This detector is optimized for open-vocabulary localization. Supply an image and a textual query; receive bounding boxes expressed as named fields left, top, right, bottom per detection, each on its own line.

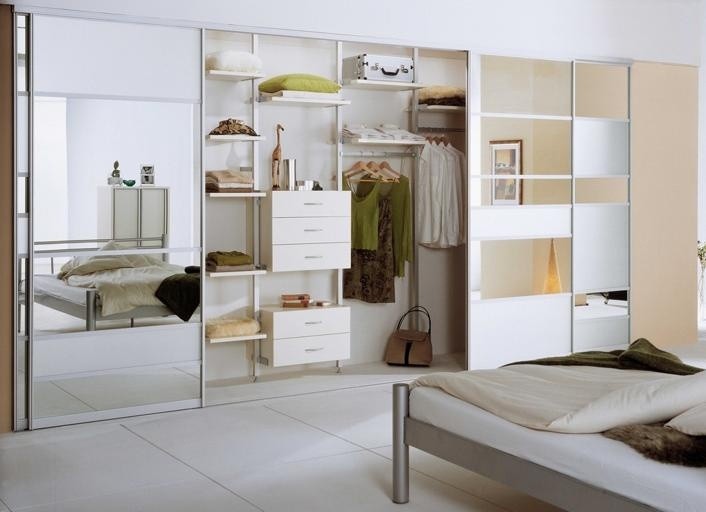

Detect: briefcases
left=342, top=54, right=414, bottom=82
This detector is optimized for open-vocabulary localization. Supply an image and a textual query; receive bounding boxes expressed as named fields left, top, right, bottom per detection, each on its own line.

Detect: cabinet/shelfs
left=97, top=185, right=170, bottom=267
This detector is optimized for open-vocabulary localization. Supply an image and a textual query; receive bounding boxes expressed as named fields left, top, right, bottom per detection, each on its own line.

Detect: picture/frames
left=490, top=140, right=524, bottom=208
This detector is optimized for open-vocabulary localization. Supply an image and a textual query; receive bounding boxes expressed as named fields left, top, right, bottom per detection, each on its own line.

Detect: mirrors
left=30, top=96, right=202, bottom=418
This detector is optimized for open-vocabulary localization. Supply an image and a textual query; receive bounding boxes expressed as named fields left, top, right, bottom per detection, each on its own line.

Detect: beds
left=29, top=238, right=199, bottom=330
left=392, top=339, right=705, bottom=512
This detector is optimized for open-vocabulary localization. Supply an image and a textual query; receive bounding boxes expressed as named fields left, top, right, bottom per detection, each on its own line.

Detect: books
left=282, top=297, right=313, bottom=304
left=310, top=299, right=332, bottom=306
left=282, top=303, right=314, bottom=308
left=280, top=294, right=310, bottom=300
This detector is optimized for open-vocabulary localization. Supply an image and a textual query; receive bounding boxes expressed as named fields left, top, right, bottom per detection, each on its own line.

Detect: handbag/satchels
left=385, top=307, right=432, bottom=367
